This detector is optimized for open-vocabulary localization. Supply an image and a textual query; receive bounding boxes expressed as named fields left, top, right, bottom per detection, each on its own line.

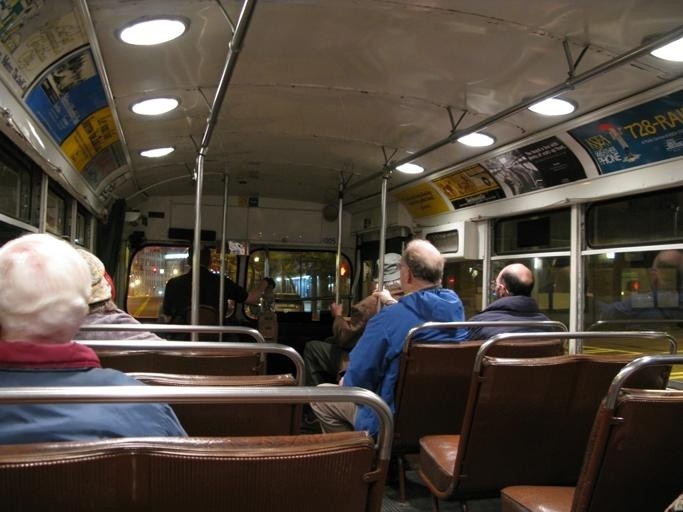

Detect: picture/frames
left=24, top=45, right=128, bottom=191
left=394, top=182, right=450, bottom=220
left=431, top=164, right=508, bottom=211
left=482, top=136, right=588, bottom=197
left=566, top=90, right=683, bottom=176
left=0, top=1, right=90, bottom=98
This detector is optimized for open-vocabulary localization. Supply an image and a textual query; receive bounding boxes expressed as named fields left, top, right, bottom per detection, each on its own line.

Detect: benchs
left=76, top=337, right=305, bottom=437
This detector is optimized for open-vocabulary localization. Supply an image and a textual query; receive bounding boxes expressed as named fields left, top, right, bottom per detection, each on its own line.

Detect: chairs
left=499, top=358, right=682, bottom=512
left=418, top=330, right=676, bottom=512
left=80, top=318, right=271, bottom=374
left=0, top=383, right=396, bottom=509
left=182, top=304, right=219, bottom=343
left=393, top=321, right=577, bottom=504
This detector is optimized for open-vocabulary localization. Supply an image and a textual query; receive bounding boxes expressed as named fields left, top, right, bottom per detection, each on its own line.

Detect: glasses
left=490, top=280, right=509, bottom=297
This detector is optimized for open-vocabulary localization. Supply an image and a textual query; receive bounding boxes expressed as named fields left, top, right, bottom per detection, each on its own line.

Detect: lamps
left=394, top=162, right=423, bottom=177
left=129, top=93, right=181, bottom=115
left=139, top=144, right=176, bottom=158
left=455, top=129, right=495, bottom=149
left=114, top=15, right=190, bottom=46
left=524, top=94, right=578, bottom=117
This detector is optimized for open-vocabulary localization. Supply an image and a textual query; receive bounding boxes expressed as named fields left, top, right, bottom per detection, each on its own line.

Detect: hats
left=75, top=248, right=113, bottom=305
left=373, top=253, right=402, bottom=283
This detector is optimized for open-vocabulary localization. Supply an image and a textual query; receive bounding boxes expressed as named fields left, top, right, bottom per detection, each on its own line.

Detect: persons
left=302, top=253, right=404, bottom=387
left=162, top=243, right=262, bottom=341
left=0, top=233, right=190, bottom=445
left=593, top=249, right=683, bottom=333
left=75, top=247, right=161, bottom=341
left=467, top=263, right=556, bottom=340
left=307, top=239, right=467, bottom=447
